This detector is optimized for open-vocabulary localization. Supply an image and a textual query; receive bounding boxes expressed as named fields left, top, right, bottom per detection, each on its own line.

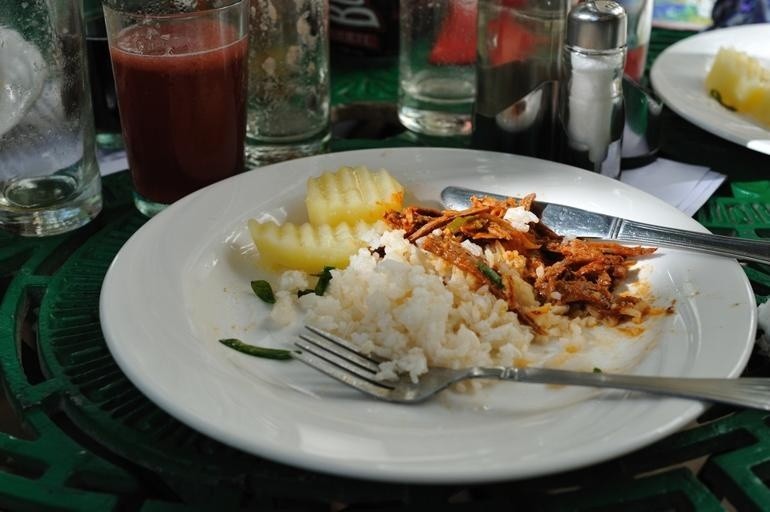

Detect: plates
left=99, top=145, right=757, bottom=484
left=649, top=22, right=770, bottom=156
left=0, top=29, right=47, bottom=137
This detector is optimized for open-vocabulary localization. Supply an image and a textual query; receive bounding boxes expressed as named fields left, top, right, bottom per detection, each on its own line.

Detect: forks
left=291, top=325, right=770, bottom=411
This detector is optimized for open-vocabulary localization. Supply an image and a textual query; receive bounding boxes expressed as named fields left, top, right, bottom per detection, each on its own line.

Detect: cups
left=102, top=0, right=250, bottom=219
left=397, top=0, right=569, bottom=160
left=0, top=0, right=103, bottom=237
left=244, top=0, right=333, bottom=170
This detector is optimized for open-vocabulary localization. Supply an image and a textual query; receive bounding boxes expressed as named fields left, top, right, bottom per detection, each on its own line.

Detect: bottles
left=567, top=0, right=653, bottom=181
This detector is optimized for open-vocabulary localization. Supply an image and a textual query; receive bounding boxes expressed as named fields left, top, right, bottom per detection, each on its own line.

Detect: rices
left=271, top=207, right=598, bottom=395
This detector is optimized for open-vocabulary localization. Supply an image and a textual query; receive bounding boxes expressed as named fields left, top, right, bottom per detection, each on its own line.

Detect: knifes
left=439, top=186, right=770, bottom=265
